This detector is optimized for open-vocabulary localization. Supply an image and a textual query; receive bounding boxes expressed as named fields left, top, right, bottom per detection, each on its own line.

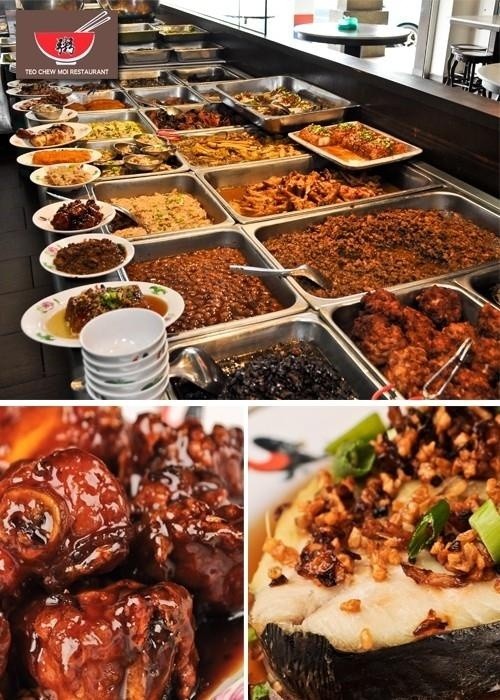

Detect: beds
left=293, top=23, right=411, bottom=58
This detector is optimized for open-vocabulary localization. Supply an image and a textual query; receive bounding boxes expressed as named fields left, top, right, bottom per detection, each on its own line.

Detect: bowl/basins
left=92, top=131, right=177, bottom=171
left=81, top=307, right=170, bottom=400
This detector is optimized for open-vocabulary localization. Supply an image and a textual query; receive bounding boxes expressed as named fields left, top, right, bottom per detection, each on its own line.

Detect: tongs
left=422, top=337, right=477, bottom=399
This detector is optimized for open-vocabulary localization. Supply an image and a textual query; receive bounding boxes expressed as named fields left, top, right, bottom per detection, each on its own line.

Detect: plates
left=28, top=162, right=102, bottom=189
left=5, top=77, right=77, bottom=124
left=9, top=122, right=92, bottom=151
left=34, top=229, right=134, bottom=278
left=31, top=200, right=117, bottom=235
left=19, top=277, right=191, bottom=351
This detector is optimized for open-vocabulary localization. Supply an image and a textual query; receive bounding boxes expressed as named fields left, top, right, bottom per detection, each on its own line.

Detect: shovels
left=230, top=264, right=329, bottom=290
left=135, top=98, right=183, bottom=115
left=236, top=104, right=289, bottom=113
left=46, top=192, right=138, bottom=226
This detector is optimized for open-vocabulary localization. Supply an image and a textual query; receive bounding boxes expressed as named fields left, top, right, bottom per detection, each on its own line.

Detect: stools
left=477, top=63, right=500, bottom=101
left=444, top=44, right=478, bottom=85
left=455, top=51, right=493, bottom=92
left=451, top=46, right=486, bottom=87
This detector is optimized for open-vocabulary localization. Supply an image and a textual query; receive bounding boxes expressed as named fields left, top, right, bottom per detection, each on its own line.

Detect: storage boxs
left=329, top=10, right=388, bottom=57
left=337, top=0, right=383, bottom=10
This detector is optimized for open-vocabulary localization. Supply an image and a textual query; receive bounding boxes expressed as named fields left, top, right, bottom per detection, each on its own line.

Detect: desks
left=450, top=15, right=500, bottom=32
